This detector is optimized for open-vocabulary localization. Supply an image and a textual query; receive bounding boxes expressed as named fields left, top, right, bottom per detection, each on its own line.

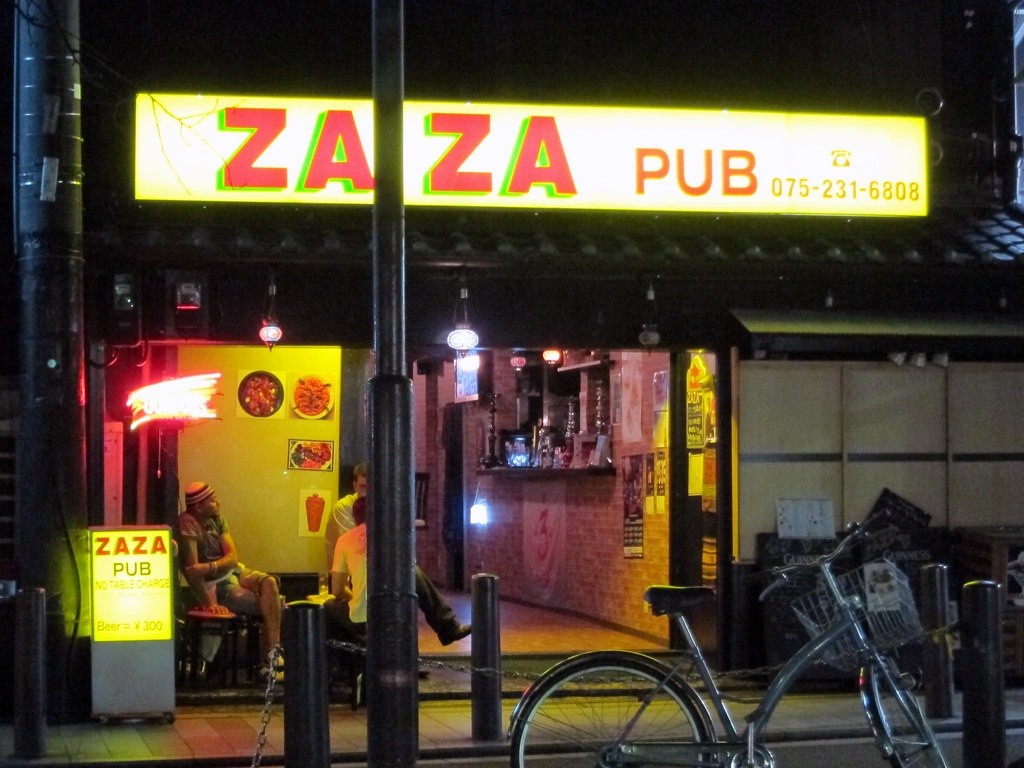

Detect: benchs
left=268, top=570, right=320, bottom=605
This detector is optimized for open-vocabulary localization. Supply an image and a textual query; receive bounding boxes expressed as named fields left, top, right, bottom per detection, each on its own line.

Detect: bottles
left=305, top=494, right=325, bottom=532
left=553, top=447, right=562, bottom=468
left=541, top=449, right=552, bottom=468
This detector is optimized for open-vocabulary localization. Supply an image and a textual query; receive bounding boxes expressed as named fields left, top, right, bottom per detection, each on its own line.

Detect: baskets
left=789, top=558, right=922, bottom=671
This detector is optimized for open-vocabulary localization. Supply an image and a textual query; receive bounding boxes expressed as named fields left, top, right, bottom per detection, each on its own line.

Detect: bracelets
left=208, top=561, right=218, bottom=579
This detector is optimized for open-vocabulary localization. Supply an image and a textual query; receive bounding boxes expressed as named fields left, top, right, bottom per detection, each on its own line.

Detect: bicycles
left=506, top=508, right=948, bottom=768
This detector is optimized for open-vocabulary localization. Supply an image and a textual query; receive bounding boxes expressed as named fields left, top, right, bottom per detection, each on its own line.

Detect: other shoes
left=441, top=624, right=472, bottom=646
left=418, top=669, right=429, bottom=677
left=266, top=649, right=284, bottom=668
left=259, top=665, right=284, bottom=681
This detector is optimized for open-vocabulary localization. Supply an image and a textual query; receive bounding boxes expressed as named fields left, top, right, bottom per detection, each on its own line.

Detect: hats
left=185, top=482, right=214, bottom=505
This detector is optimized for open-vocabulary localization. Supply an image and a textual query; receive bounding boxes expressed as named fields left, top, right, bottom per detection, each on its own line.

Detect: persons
left=322, top=495, right=366, bottom=671
left=177, top=481, right=286, bottom=682
left=324, top=462, right=472, bottom=646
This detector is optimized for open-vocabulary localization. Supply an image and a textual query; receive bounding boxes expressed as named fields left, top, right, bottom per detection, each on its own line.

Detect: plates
left=290, top=374, right=334, bottom=419
left=238, top=371, right=283, bottom=416
left=290, top=442, right=331, bottom=469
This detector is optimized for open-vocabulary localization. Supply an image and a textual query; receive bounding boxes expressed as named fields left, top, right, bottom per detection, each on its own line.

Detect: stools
left=186, top=609, right=240, bottom=688
left=346, top=628, right=368, bottom=711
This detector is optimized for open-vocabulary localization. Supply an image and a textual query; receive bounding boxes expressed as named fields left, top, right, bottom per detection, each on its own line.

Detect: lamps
left=640, top=288, right=661, bottom=355
left=257, top=283, right=283, bottom=347
left=446, top=264, right=480, bottom=355
left=175, top=264, right=206, bottom=310
